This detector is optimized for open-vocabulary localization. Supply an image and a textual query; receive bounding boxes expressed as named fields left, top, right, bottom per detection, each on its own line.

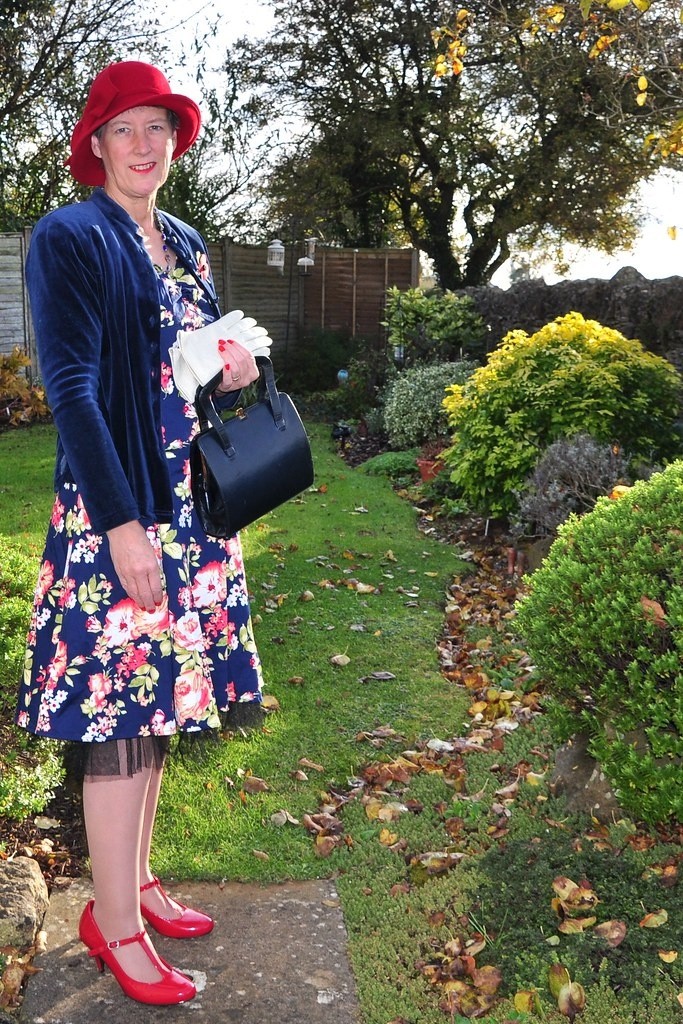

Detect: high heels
left=78, top=899, right=197, bottom=1007
left=139, top=875, right=215, bottom=939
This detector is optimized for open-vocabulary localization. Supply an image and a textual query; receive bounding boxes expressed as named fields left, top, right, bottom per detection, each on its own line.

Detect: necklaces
left=153, top=215, right=171, bottom=273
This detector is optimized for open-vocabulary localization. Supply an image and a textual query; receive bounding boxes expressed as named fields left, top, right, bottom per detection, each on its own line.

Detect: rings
left=233, top=375, right=240, bottom=381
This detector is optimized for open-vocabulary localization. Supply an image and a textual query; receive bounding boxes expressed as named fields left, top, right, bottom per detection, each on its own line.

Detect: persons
left=14, top=62, right=267, bottom=1005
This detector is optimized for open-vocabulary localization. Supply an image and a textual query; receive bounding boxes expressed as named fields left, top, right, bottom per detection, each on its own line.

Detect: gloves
left=176, top=309, right=273, bottom=388
left=167, top=346, right=201, bottom=405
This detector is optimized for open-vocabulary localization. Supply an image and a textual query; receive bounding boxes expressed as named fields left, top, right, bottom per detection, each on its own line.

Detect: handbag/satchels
left=189, top=355, right=315, bottom=541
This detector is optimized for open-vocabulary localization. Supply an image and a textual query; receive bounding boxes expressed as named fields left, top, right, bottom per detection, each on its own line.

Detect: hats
left=63, top=60, right=202, bottom=186
